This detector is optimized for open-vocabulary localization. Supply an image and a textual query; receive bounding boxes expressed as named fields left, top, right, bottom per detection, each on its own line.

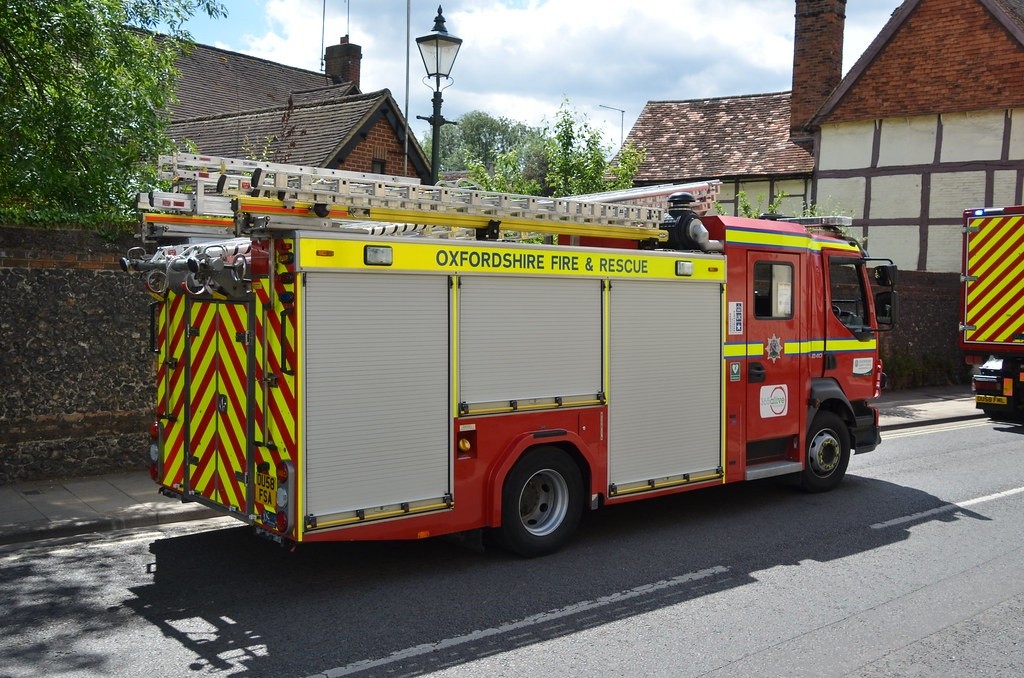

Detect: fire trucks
left=117, top=148, right=899, bottom=558
left=958, top=205, right=1024, bottom=420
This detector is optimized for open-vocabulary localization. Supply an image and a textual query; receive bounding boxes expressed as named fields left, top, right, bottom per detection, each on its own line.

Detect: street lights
left=415, top=4, right=464, bottom=188
